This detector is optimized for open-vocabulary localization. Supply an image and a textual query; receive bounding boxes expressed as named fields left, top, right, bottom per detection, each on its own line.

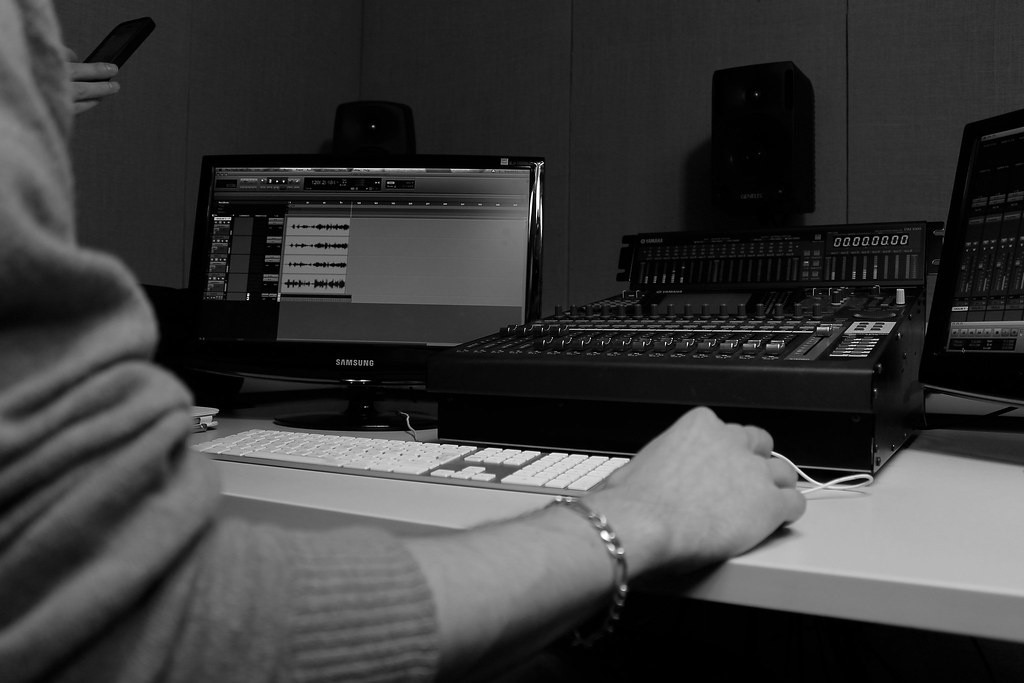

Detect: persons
left=0, top=0, right=807, bottom=683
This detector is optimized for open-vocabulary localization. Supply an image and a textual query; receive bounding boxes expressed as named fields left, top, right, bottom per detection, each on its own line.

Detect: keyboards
left=192, top=428, right=632, bottom=498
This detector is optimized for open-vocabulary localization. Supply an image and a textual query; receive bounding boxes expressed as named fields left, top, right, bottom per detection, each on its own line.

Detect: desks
left=190, top=379, right=1024, bottom=643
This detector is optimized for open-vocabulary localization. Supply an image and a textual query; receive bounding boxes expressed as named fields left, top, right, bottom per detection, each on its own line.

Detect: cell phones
left=81, top=17, right=157, bottom=82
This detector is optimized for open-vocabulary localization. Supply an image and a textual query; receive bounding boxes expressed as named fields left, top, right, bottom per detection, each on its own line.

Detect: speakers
left=713, top=60, right=818, bottom=214
left=336, top=100, right=418, bottom=158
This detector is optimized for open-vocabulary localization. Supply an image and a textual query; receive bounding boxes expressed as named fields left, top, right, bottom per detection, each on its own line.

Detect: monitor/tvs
left=919, top=110, right=1024, bottom=407
left=184, top=156, right=547, bottom=431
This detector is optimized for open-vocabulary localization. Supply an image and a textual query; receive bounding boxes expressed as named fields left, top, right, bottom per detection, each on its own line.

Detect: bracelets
left=544, top=497, right=629, bottom=647
